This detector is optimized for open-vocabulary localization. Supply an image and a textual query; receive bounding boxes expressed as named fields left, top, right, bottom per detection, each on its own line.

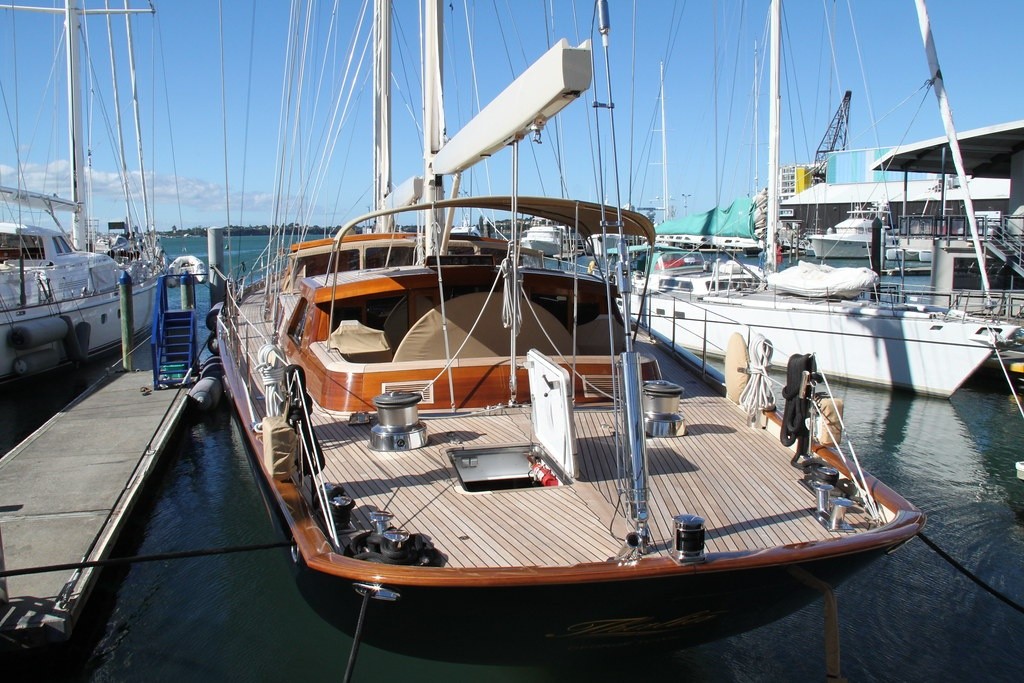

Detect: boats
left=806, top=205, right=883, bottom=260
left=513, top=213, right=578, bottom=261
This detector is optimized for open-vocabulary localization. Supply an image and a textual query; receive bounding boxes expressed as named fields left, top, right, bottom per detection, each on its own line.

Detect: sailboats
left=198, top=0, right=923, bottom=604
left=617, top=0, right=1017, bottom=401
left=0, top=0, right=173, bottom=392
